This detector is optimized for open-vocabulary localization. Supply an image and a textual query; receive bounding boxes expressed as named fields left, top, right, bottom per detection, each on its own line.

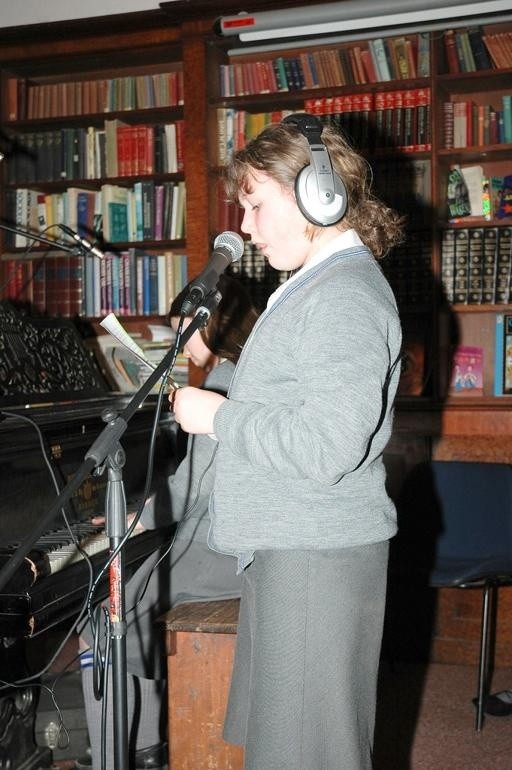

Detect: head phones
left=281, top=113, right=347, bottom=227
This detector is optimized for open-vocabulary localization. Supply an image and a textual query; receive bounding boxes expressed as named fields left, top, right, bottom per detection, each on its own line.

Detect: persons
left=164, top=108, right=401, bottom=770
left=68, top=267, right=267, bottom=770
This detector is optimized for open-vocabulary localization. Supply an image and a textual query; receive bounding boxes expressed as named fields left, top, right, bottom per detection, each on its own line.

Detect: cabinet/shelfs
left=2, top=20, right=207, bottom=389
left=209, top=32, right=511, bottom=463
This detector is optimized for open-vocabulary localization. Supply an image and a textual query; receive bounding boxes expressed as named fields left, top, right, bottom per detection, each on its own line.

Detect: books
left=1, top=71, right=189, bottom=182
left=397, top=313, right=512, bottom=398
left=217, top=174, right=298, bottom=287
left=440, top=28, right=509, bottom=306
left=98, top=326, right=189, bottom=392
left=375, top=157, right=434, bottom=305
left=218, top=34, right=431, bottom=164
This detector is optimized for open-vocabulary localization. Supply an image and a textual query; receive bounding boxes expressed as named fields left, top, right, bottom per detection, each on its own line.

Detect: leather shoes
left=74, top=743, right=164, bottom=770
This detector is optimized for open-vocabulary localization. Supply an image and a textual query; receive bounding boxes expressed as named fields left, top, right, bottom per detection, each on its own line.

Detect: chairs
left=405, top=464, right=510, bottom=732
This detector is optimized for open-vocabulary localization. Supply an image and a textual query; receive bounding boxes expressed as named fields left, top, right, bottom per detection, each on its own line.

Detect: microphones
left=59, top=223, right=105, bottom=259
left=181, top=230, right=244, bottom=315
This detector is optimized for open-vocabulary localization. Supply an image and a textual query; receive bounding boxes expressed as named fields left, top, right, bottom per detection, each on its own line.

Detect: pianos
left=1, top=298, right=189, bottom=770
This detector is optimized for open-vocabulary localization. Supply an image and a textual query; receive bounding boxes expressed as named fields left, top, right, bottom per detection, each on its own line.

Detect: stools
left=152, top=597, right=245, bottom=770
